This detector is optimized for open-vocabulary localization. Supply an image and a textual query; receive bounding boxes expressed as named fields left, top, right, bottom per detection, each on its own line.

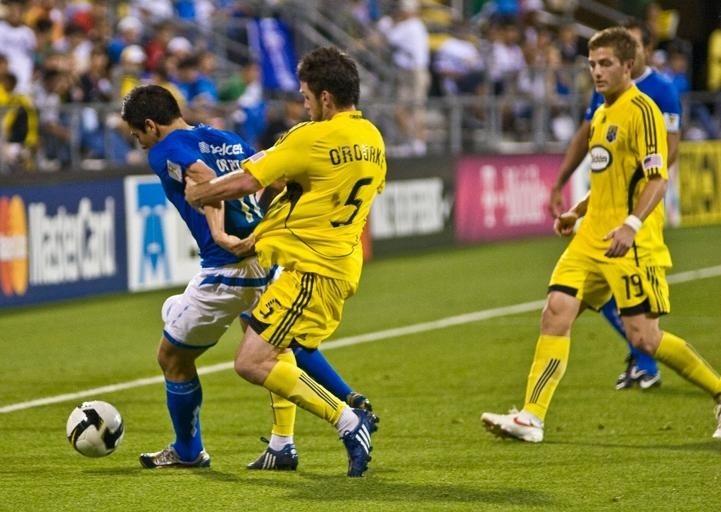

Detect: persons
left=479, top=22, right=721, bottom=446
left=547, top=17, right=685, bottom=394
left=181, top=44, right=389, bottom=479
left=0, top=1, right=721, bottom=178
left=121, top=82, right=376, bottom=470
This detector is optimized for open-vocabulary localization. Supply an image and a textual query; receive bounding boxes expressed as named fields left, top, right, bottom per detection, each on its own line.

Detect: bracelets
left=621, top=213, right=644, bottom=234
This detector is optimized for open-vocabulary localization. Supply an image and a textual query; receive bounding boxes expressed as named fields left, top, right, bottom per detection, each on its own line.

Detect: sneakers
left=340, top=407, right=380, bottom=477
left=481, top=408, right=544, bottom=443
left=139, top=443, right=211, bottom=468
left=712, top=411, right=721, bottom=439
left=246, top=444, right=298, bottom=471
left=349, top=393, right=372, bottom=413
left=615, top=366, right=662, bottom=392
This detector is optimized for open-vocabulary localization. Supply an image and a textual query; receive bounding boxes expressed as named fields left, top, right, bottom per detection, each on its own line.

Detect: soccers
left=66, top=401, right=124, bottom=457
